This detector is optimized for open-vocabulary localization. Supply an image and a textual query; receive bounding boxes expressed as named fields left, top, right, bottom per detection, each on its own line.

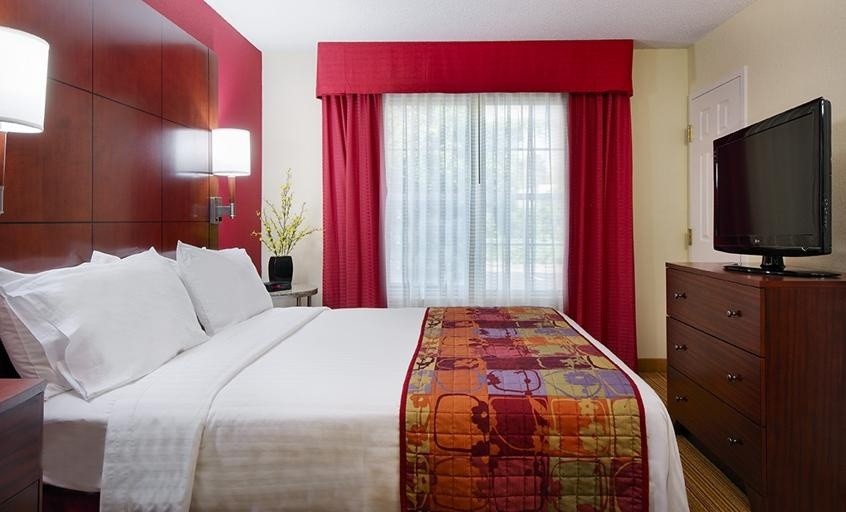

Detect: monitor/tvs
left=712, top=95, right=843, bottom=277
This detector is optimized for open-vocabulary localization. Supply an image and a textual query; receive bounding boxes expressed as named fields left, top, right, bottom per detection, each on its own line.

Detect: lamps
left=0, top=25, right=50, bottom=134
left=210, top=127, right=251, bottom=224
left=174, top=127, right=212, bottom=175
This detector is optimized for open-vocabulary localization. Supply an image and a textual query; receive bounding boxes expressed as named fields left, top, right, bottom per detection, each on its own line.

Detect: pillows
left=0, top=249, right=123, bottom=402
left=176, top=239, right=274, bottom=337
left=0, top=247, right=210, bottom=401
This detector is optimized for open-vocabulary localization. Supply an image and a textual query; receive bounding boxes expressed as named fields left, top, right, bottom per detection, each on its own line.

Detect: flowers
left=250, top=166, right=324, bottom=256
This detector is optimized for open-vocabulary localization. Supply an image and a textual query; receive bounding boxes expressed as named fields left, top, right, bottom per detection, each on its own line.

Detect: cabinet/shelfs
left=664, top=260, right=845, bottom=512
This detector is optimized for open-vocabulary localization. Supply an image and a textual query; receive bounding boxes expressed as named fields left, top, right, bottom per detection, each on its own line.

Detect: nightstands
left=1, top=377, right=48, bottom=512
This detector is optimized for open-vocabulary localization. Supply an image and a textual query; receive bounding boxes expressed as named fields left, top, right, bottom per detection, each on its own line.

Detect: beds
left=40, top=304, right=691, bottom=512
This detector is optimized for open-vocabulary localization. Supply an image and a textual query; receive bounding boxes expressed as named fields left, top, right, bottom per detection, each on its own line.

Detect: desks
left=268, top=283, right=318, bottom=307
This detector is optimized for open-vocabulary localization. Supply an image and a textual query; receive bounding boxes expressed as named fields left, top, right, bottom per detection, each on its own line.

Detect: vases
left=267, top=257, right=293, bottom=291
